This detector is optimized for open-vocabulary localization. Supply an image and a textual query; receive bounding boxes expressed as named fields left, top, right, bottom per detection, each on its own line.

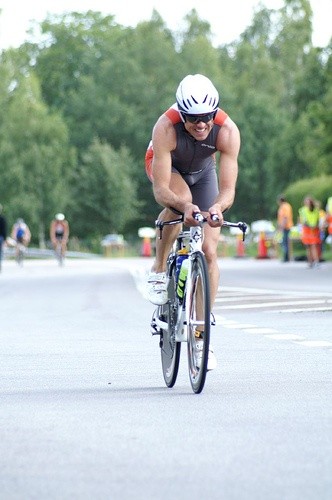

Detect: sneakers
left=147, top=268, right=168, bottom=305
left=195, top=339, right=218, bottom=370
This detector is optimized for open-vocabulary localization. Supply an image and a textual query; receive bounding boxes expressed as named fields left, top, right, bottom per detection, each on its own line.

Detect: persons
left=144, top=73, right=241, bottom=371
left=0, top=195, right=332, bottom=271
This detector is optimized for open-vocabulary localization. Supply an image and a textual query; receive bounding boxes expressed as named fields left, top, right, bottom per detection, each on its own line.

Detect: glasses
left=183, top=113, right=215, bottom=123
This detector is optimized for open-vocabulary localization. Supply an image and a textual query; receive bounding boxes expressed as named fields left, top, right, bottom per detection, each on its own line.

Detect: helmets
left=56, top=213, right=65, bottom=221
left=176, top=74, right=220, bottom=123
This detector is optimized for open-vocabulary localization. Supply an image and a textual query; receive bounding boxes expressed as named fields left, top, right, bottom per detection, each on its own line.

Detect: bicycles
left=13, top=239, right=28, bottom=265
left=150, top=212, right=248, bottom=394
left=51, top=238, right=70, bottom=274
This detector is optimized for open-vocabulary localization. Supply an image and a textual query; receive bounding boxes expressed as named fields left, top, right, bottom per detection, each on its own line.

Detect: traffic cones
left=101, top=245, right=113, bottom=262
left=140, top=235, right=156, bottom=259
left=229, top=228, right=247, bottom=261
left=255, top=231, right=270, bottom=262
left=113, top=241, right=126, bottom=259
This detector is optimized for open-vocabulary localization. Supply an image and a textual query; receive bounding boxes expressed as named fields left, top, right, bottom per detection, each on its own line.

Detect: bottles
left=176, top=249, right=189, bottom=283
left=177, top=255, right=192, bottom=297
